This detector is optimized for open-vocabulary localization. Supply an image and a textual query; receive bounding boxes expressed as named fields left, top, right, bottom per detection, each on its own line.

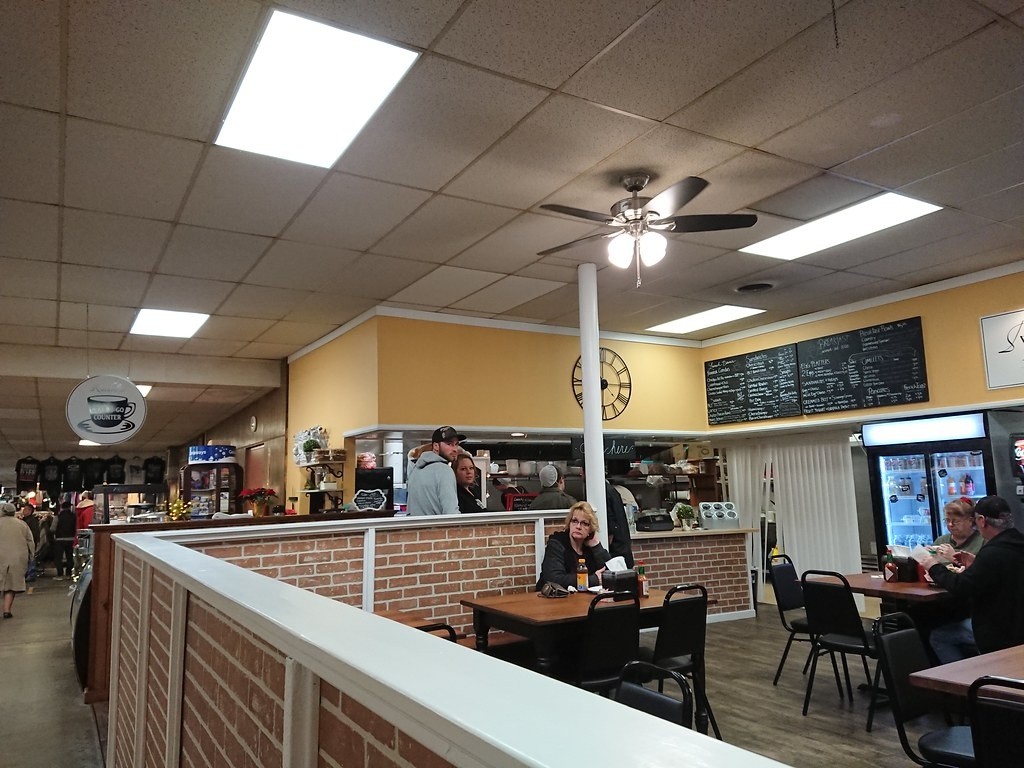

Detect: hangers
left=19, top=452, right=165, bottom=462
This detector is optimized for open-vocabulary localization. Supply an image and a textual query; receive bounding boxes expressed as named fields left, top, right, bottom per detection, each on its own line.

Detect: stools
left=394, top=615, right=466, bottom=640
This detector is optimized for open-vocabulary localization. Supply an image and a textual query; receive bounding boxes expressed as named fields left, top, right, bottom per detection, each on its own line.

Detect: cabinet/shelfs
left=421, top=437, right=717, bottom=518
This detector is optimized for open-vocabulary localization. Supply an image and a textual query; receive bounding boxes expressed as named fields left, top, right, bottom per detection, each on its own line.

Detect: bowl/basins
left=505, top=459, right=581, bottom=477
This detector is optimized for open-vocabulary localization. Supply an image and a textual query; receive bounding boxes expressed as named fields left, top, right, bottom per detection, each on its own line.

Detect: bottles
left=576, top=559, right=588, bottom=593
left=887, top=474, right=975, bottom=496
left=894, top=533, right=933, bottom=551
left=636, top=561, right=650, bottom=599
left=884, top=550, right=897, bottom=583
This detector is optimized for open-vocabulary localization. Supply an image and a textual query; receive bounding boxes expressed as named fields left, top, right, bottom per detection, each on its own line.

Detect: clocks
left=571, top=347, right=632, bottom=421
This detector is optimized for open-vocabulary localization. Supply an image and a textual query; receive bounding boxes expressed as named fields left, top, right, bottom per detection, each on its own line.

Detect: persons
left=50, top=502, right=77, bottom=580
left=451, top=454, right=487, bottom=514
left=72, top=491, right=94, bottom=546
left=528, top=464, right=577, bottom=510
left=919, top=495, right=1024, bottom=665
left=492, top=478, right=528, bottom=509
left=536, top=501, right=612, bottom=591
left=407, top=426, right=467, bottom=516
left=0, top=503, right=35, bottom=618
left=579, top=461, right=634, bottom=569
left=21, top=503, right=41, bottom=594
left=924, top=497, right=990, bottom=568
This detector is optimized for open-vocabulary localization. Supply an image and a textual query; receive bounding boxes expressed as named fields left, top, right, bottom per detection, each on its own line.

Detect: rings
left=941, top=550, right=943, bottom=554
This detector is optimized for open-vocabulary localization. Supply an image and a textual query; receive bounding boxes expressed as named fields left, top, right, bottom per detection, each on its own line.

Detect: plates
left=588, top=585, right=604, bottom=593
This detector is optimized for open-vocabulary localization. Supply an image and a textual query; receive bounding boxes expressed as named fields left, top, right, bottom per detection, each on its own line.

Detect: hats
left=966, top=495, right=1011, bottom=521
left=4, top=503, right=16, bottom=512
left=538, top=465, right=558, bottom=487
left=62, top=502, right=73, bottom=508
left=432, top=426, right=466, bottom=443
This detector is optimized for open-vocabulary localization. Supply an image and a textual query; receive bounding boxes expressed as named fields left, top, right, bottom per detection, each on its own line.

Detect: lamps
left=609, top=196, right=667, bottom=270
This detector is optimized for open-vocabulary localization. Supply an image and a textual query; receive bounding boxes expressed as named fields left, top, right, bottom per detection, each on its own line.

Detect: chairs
left=769, top=553, right=1024, bottom=768
left=548, top=584, right=724, bottom=741
left=414, top=623, right=456, bottom=642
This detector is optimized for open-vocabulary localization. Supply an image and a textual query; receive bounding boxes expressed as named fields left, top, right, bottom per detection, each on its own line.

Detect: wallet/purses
left=537, top=581, right=569, bottom=597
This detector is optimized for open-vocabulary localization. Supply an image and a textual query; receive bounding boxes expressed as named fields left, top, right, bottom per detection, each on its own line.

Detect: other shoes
left=67, top=575, right=71, bottom=579
left=53, top=575, right=64, bottom=580
left=3, top=611, right=13, bottom=618
left=25, top=575, right=36, bottom=582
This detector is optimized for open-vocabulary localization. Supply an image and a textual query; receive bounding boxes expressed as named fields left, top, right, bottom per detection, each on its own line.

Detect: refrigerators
left=861, top=409, right=1024, bottom=572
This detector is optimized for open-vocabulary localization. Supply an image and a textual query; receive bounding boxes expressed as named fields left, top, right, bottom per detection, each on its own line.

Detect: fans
left=536, top=172, right=759, bottom=288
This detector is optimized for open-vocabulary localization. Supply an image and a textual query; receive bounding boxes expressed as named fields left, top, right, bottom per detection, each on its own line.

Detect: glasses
left=570, top=517, right=591, bottom=529
left=943, top=517, right=970, bottom=525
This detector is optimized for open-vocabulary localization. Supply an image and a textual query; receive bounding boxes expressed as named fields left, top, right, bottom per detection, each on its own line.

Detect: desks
left=794, top=572, right=958, bottom=695
left=909, top=644, right=1024, bottom=703
left=460, top=588, right=719, bottom=736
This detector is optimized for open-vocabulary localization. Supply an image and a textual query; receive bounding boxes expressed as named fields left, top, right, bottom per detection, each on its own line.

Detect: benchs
left=456, top=629, right=530, bottom=658
